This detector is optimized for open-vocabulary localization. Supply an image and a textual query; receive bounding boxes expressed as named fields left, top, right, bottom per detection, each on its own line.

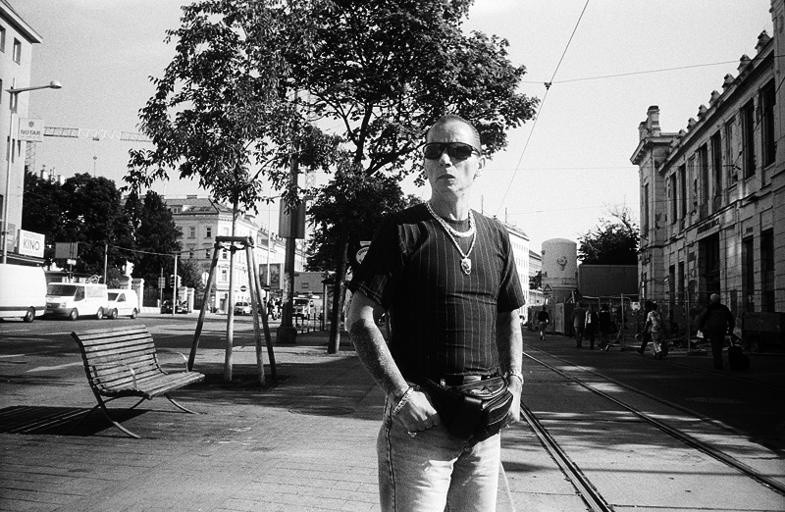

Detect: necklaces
left=425, top=200, right=477, bottom=276
left=427, top=201, right=475, bottom=237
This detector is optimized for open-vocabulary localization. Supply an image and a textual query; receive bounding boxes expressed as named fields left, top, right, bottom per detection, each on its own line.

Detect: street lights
left=1, top=80, right=62, bottom=265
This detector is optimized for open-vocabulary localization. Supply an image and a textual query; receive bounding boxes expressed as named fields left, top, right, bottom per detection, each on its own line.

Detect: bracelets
left=504, top=370, right=524, bottom=385
left=392, top=387, right=414, bottom=417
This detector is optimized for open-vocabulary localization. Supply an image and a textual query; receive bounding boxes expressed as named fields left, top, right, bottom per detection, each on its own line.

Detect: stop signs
left=240, top=286, right=246, bottom=291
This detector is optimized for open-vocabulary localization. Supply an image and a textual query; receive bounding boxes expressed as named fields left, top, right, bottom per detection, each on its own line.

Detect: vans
left=0, top=263, right=49, bottom=322
left=46, top=279, right=109, bottom=320
left=291, top=297, right=315, bottom=315
left=109, top=288, right=140, bottom=319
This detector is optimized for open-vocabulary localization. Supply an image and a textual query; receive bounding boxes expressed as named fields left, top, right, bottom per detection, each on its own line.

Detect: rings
left=408, top=432, right=418, bottom=438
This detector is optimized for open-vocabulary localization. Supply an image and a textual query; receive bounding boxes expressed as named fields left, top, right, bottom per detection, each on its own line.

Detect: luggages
left=729, top=347, right=749, bottom=370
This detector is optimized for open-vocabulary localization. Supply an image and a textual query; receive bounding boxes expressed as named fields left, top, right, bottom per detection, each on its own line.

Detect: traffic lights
left=170, top=274, right=181, bottom=288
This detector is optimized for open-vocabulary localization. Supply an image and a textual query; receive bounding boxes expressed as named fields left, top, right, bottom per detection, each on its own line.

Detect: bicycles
left=539, top=324, right=545, bottom=340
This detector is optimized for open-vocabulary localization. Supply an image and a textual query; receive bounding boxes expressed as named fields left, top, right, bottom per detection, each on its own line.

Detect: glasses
left=423, top=142, right=480, bottom=160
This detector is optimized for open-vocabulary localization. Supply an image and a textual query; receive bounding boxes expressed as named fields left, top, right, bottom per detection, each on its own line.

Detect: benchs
left=70, top=324, right=206, bottom=440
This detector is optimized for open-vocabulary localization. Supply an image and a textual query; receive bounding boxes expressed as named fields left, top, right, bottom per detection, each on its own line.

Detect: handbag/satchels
left=425, top=375, right=513, bottom=440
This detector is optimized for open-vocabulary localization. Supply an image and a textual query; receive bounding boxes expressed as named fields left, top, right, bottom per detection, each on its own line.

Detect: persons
left=570, top=300, right=668, bottom=359
left=696, top=293, right=735, bottom=370
left=266, top=297, right=283, bottom=320
left=346, top=115, right=527, bottom=512
left=537, top=307, right=552, bottom=341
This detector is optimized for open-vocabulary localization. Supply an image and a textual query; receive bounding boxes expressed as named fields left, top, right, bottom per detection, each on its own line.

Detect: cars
left=235, top=301, right=253, bottom=315
left=161, top=298, right=189, bottom=313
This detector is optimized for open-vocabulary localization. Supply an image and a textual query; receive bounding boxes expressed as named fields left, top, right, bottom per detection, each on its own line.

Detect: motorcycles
left=648, top=333, right=668, bottom=359
